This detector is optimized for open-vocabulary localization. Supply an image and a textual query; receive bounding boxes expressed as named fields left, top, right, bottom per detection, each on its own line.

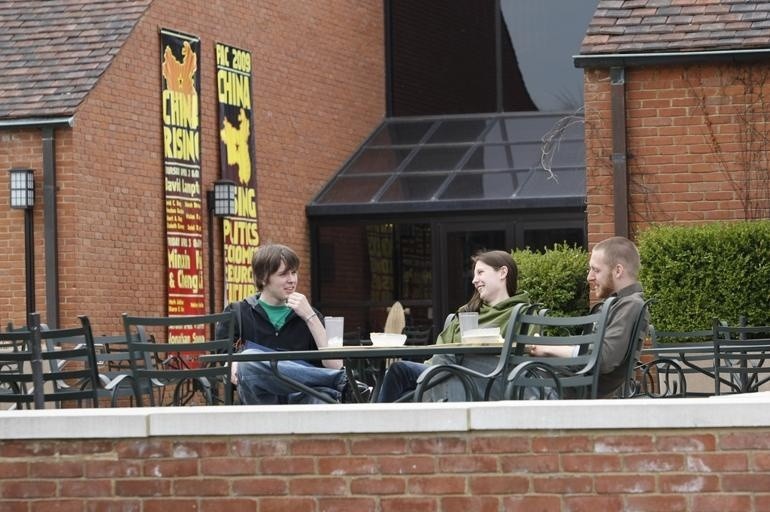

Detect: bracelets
left=304, top=312, right=318, bottom=323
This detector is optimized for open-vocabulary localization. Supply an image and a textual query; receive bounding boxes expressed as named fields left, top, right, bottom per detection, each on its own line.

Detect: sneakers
left=343, top=380, right=373, bottom=401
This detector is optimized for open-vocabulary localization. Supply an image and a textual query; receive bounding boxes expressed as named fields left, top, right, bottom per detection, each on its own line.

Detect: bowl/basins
left=370, top=332, right=407, bottom=347
left=461, top=328, right=501, bottom=344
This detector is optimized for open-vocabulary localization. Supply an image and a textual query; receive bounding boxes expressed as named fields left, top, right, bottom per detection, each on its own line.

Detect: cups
left=323, top=316, right=344, bottom=348
left=457, top=313, right=479, bottom=335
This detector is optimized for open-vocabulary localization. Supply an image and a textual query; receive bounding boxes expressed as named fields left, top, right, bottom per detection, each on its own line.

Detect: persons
left=206, top=242, right=372, bottom=404
left=375, top=250, right=540, bottom=402
left=517, top=236, right=651, bottom=401
